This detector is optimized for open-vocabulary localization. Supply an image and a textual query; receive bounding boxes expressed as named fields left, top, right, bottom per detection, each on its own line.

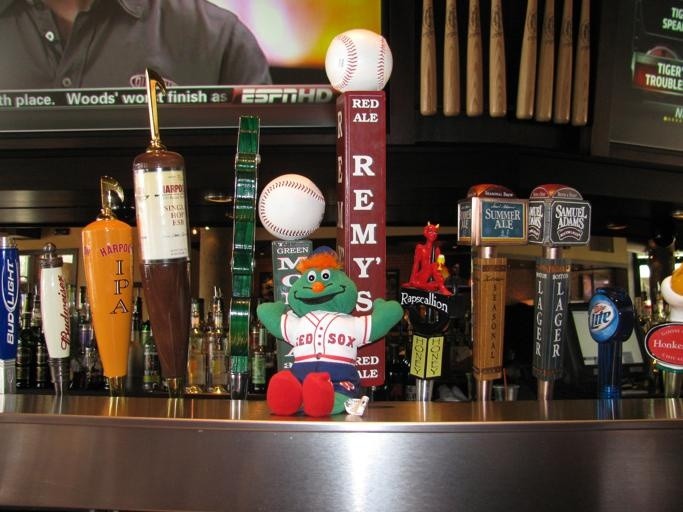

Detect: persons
left=2, top=2, right=276, bottom=89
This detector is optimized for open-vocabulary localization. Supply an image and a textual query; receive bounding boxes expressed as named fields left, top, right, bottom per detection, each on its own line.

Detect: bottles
left=79, top=175, right=138, bottom=395
left=131, top=66, right=191, bottom=396
left=35, top=239, right=77, bottom=393
left=15, top=287, right=275, bottom=397
left=1, top=235, right=21, bottom=395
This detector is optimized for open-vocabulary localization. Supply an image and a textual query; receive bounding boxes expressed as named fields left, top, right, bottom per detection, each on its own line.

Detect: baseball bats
left=516, top=0, right=591, bottom=127
left=419, top=0, right=507, bottom=118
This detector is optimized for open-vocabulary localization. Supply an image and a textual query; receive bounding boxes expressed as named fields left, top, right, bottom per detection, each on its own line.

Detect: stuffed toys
left=255, top=245, right=405, bottom=417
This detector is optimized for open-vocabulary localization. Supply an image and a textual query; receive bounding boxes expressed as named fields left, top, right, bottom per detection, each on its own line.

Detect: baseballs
left=258, top=174, right=326, bottom=241
left=324, top=27, right=394, bottom=92
